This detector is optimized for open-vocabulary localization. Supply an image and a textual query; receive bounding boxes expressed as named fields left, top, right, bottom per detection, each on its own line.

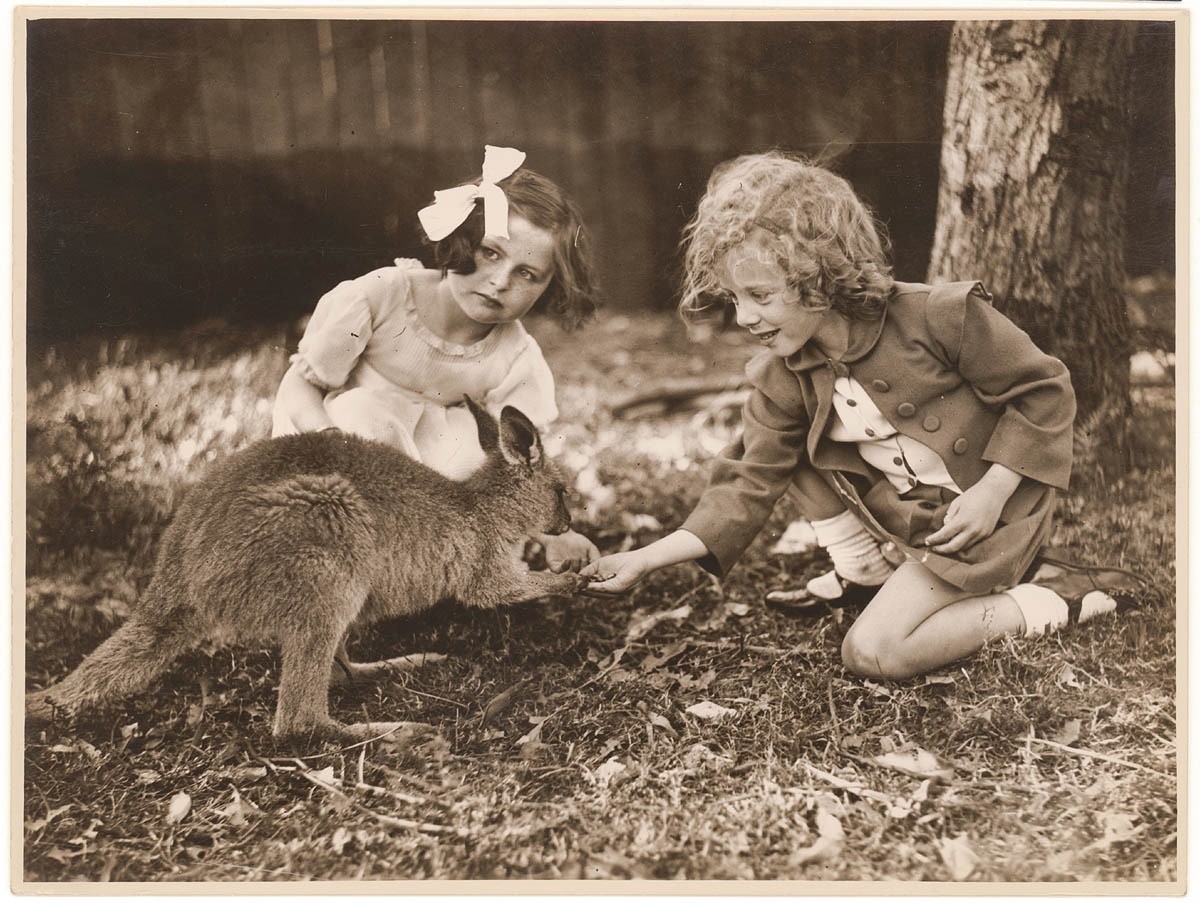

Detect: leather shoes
left=1029, top=546, right=1166, bottom=627
left=766, top=566, right=888, bottom=614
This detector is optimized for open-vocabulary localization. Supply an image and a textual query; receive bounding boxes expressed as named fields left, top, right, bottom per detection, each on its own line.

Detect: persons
left=581, top=151, right=1168, bottom=680
left=270, top=144, right=607, bottom=481
